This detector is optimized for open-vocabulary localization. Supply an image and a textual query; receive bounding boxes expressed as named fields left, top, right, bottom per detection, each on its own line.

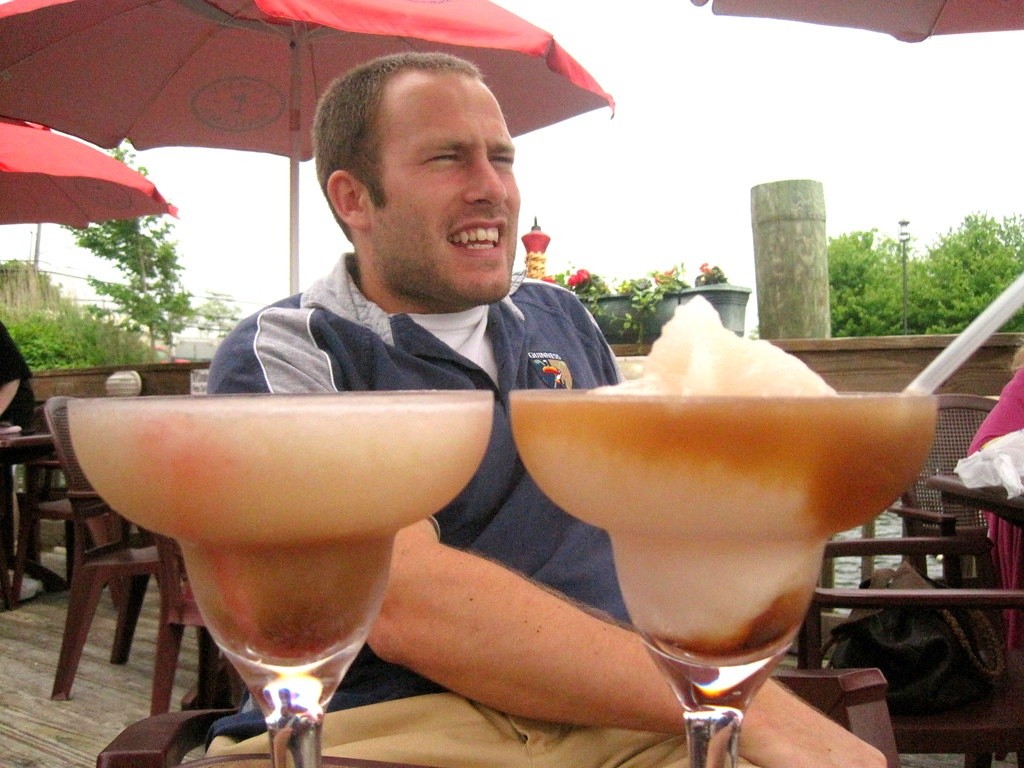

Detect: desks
left=28, top=359, right=211, bottom=554
left=924, top=473, right=1024, bottom=586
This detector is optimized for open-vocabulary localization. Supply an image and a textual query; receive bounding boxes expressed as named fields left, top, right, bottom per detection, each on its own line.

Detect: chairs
left=94, top=667, right=901, bottom=767
left=9, top=405, right=133, bottom=610
left=42, top=395, right=159, bottom=698
left=800, top=535, right=1024, bottom=767
left=151, top=534, right=234, bottom=716
left=887, top=390, right=1002, bottom=586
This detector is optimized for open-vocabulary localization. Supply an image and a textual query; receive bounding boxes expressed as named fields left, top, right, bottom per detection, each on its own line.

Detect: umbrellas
left=2, top=116, right=181, bottom=229
left=1, top=0, right=620, bottom=297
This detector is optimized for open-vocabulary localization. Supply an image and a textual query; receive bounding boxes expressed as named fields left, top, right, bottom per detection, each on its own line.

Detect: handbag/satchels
left=826, top=559, right=1007, bottom=716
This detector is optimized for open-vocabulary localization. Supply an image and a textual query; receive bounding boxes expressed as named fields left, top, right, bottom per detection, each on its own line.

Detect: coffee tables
left=0, top=434, right=55, bottom=612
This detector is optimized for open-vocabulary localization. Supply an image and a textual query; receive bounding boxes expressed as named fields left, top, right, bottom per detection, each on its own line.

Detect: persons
left=961, top=346, right=1022, bottom=652
left=183, top=48, right=892, bottom=768
left=0, top=320, right=36, bottom=434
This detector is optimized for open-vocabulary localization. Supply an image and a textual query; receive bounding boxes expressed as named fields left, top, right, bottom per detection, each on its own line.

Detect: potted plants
left=641, top=264, right=688, bottom=342
left=541, top=269, right=595, bottom=316
left=587, top=279, right=644, bottom=343
left=674, top=263, right=751, bottom=336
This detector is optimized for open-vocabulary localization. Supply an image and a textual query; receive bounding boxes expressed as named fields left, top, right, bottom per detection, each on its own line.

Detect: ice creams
left=510, top=293, right=940, bottom=658
